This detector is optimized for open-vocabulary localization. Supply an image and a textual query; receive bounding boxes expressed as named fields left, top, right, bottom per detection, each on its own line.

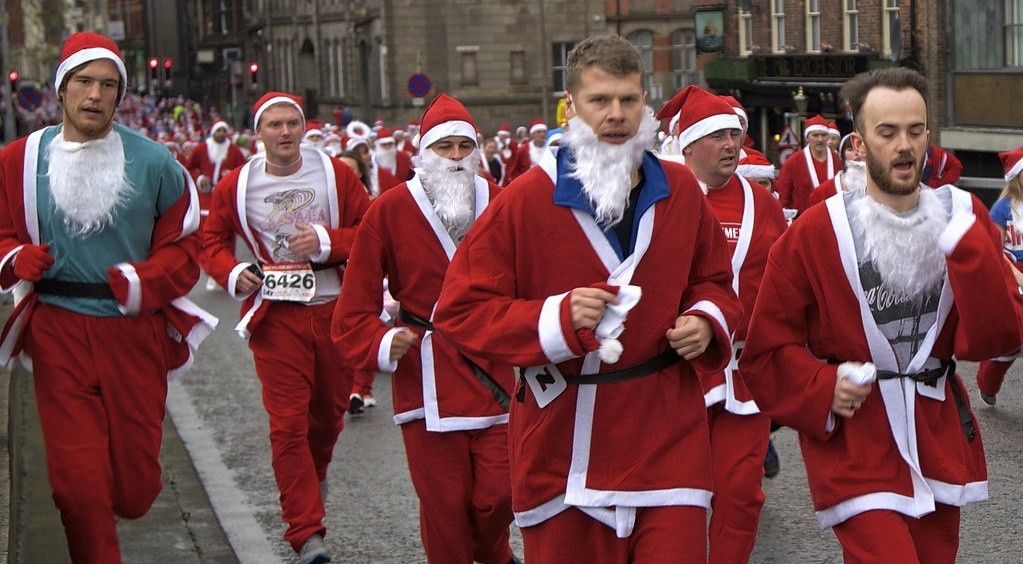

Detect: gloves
left=107, top=268, right=128, bottom=306
left=9, top=243, right=54, bottom=281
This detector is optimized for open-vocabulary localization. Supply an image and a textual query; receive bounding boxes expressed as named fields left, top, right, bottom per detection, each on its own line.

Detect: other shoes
left=349, top=393, right=364, bottom=416
left=363, top=397, right=378, bottom=409
left=764, top=439, right=780, bottom=480
left=978, top=385, right=998, bottom=407
left=205, top=276, right=218, bottom=292
left=298, top=530, right=332, bottom=564
left=318, top=479, right=330, bottom=505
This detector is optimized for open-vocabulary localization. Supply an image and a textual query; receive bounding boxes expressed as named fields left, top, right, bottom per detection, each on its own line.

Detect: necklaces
left=266, top=155, right=301, bottom=169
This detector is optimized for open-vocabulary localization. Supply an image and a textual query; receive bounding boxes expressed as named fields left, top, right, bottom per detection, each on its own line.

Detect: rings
left=849, top=402, right=855, bottom=409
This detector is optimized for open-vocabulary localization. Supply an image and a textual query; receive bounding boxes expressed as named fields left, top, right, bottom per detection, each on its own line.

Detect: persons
left=0, top=32, right=201, bottom=564
left=973, top=147, right=1023, bottom=406
left=475, top=118, right=548, bottom=186
left=300, top=118, right=423, bottom=414
left=739, top=66, right=1023, bottom=563
left=921, top=146, right=962, bottom=189
left=198, top=91, right=375, bottom=563
left=6, top=85, right=265, bottom=293
left=777, top=114, right=867, bottom=214
left=657, top=86, right=800, bottom=564
left=330, top=94, right=523, bottom=563
left=431, top=35, right=746, bottom=564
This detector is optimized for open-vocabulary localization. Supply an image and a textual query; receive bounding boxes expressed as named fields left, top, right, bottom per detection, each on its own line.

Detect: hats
left=54, top=30, right=127, bottom=109
left=999, top=146, right=1023, bottom=182
left=589, top=283, right=642, bottom=363
left=805, top=114, right=830, bottom=138
left=171, top=108, right=554, bottom=169
left=825, top=121, right=840, bottom=137
left=417, top=93, right=479, bottom=159
left=732, top=151, right=774, bottom=179
left=252, top=91, right=307, bottom=134
left=650, top=85, right=748, bottom=157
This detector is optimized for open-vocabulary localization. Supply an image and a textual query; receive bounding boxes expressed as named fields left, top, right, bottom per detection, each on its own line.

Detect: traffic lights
left=248, top=63, right=259, bottom=85
left=164, top=60, right=172, bottom=81
left=149, top=58, right=157, bottom=81
left=771, top=115, right=781, bottom=144
left=10, top=71, right=18, bottom=92
